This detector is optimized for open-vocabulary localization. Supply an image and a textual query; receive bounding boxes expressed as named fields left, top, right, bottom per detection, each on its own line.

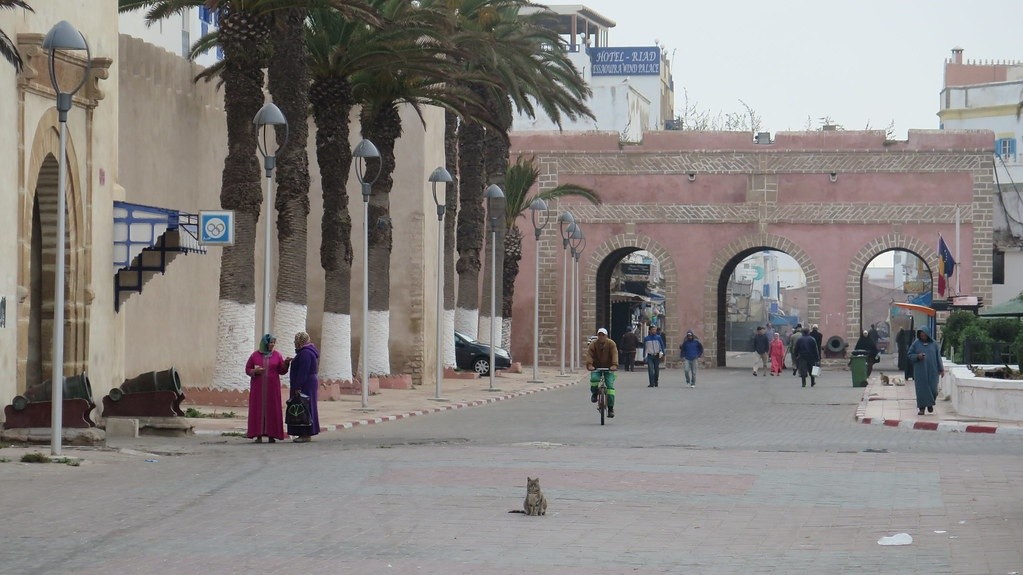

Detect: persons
left=868, top=324, right=881, bottom=349
left=680, top=333, right=704, bottom=387
left=586, top=328, right=618, bottom=418
left=906, top=325, right=944, bottom=415
left=643, top=326, right=665, bottom=387
left=894, top=327, right=907, bottom=370
left=794, top=327, right=820, bottom=387
left=854, top=330, right=878, bottom=378
left=679, top=329, right=700, bottom=350
left=286, top=332, right=320, bottom=443
left=245, top=334, right=292, bottom=443
left=656, top=327, right=666, bottom=350
left=752, top=322, right=823, bottom=376
left=619, top=326, right=639, bottom=372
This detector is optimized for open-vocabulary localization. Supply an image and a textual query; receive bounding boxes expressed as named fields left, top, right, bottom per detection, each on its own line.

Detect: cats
left=508, top=477, right=546, bottom=517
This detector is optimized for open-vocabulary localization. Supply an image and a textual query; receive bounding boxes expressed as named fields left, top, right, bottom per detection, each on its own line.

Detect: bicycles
left=590, top=367, right=618, bottom=426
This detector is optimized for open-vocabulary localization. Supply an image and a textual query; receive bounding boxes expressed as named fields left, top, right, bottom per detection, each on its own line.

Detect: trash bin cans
left=850, top=356, right=868, bottom=387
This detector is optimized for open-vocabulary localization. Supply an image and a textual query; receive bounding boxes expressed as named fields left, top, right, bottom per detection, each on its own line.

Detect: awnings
left=609, top=291, right=651, bottom=304
left=892, top=302, right=935, bottom=317
left=112, top=200, right=206, bottom=314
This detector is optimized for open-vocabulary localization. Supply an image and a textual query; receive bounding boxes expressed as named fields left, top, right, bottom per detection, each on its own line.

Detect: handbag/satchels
left=659, top=351, right=663, bottom=359
left=811, top=365, right=822, bottom=377
left=284, top=393, right=312, bottom=426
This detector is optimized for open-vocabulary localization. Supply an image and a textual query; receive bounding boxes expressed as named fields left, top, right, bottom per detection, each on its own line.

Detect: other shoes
left=293, top=435, right=311, bottom=442
left=269, top=438, right=275, bottom=443
left=255, top=438, right=262, bottom=442
left=776, top=374, right=779, bottom=376
left=753, top=372, right=757, bottom=376
left=927, top=406, right=933, bottom=413
left=918, top=408, right=925, bottom=415
left=691, top=385, right=695, bottom=388
left=793, top=373, right=796, bottom=376
left=811, top=383, right=816, bottom=387
left=648, top=385, right=654, bottom=387
left=763, top=373, right=766, bottom=376
left=608, top=406, right=614, bottom=417
left=591, top=394, right=597, bottom=403
left=802, top=385, right=805, bottom=387
left=655, top=381, right=658, bottom=387
left=687, top=383, right=690, bottom=387
left=769, top=372, right=774, bottom=376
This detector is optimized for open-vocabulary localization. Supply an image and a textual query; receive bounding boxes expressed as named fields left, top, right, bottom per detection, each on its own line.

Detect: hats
left=597, top=328, right=607, bottom=335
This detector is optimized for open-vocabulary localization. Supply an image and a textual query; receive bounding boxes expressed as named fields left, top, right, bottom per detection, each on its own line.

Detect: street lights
left=557, top=211, right=588, bottom=378
left=428, top=166, right=455, bottom=404
left=250, top=91, right=290, bottom=346
left=351, top=135, right=385, bottom=410
left=39, top=19, right=98, bottom=456
left=528, top=196, right=549, bottom=385
left=482, top=184, right=505, bottom=393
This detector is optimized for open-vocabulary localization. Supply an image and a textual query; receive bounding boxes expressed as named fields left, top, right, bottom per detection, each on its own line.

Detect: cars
left=452, top=331, right=512, bottom=378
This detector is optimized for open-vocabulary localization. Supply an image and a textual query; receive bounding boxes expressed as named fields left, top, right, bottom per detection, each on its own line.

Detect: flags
left=938, top=236, right=954, bottom=297
